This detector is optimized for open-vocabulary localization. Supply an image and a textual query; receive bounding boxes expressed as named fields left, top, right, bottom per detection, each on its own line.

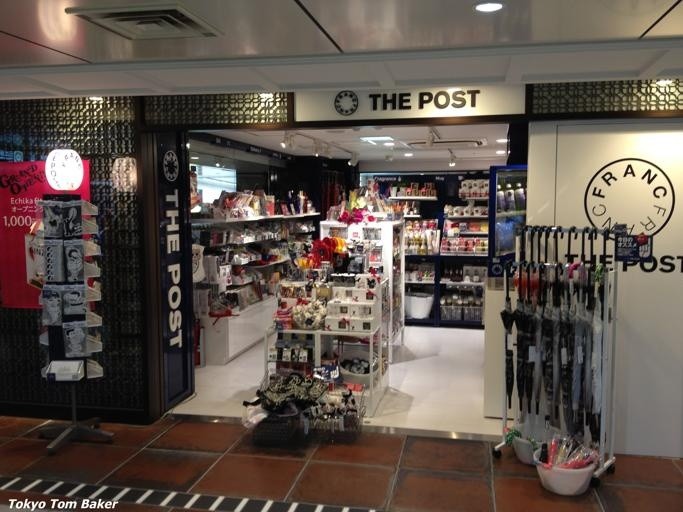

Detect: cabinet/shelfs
left=30, top=192, right=116, bottom=457
left=190, top=169, right=527, bottom=420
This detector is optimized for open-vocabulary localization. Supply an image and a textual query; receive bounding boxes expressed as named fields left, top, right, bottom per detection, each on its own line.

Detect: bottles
left=497, top=182, right=528, bottom=211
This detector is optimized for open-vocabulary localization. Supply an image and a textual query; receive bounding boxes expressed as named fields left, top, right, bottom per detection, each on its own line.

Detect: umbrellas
left=501, top=224, right=611, bottom=468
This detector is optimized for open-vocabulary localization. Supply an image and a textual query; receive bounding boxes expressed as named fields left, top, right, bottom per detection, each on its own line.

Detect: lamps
left=424, top=126, right=457, bottom=168
left=278, top=129, right=360, bottom=169
